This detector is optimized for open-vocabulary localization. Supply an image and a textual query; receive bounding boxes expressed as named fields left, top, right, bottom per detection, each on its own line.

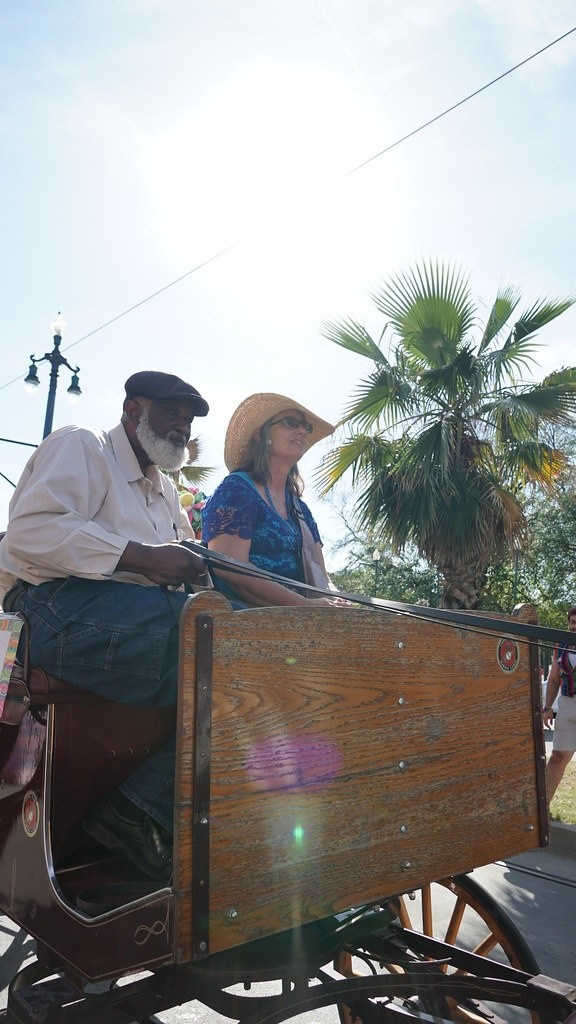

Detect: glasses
left=269, top=415, right=314, bottom=433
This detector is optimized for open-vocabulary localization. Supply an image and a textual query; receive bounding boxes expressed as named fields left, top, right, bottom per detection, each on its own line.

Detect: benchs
left=0, top=695, right=176, bottom=981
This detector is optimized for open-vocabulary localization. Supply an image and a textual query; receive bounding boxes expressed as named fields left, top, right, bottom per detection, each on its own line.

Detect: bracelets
left=543, top=707, right=553, bottom=712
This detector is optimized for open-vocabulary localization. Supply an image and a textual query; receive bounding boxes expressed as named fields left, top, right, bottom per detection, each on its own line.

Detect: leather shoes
left=83, top=795, right=173, bottom=881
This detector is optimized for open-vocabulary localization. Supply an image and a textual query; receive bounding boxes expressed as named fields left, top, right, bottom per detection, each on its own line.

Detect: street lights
left=24, top=311, right=83, bottom=443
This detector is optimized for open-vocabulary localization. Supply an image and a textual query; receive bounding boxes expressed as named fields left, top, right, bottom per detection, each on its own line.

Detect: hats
left=225, top=393, right=335, bottom=473
left=125, top=371, right=208, bottom=417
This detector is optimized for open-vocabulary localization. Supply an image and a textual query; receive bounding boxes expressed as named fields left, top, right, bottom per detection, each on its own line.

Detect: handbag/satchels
left=292, top=491, right=343, bottom=606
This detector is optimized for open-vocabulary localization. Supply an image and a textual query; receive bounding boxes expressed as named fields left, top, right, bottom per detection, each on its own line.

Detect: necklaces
left=559, top=644, right=576, bottom=675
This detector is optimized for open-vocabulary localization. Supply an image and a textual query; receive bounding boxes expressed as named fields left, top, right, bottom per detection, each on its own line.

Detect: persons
left=0, top=371, right=248, bottom=883
left=204, top=393, right=358, bottom=608
left=543, top=610, right=576, bottom=802
